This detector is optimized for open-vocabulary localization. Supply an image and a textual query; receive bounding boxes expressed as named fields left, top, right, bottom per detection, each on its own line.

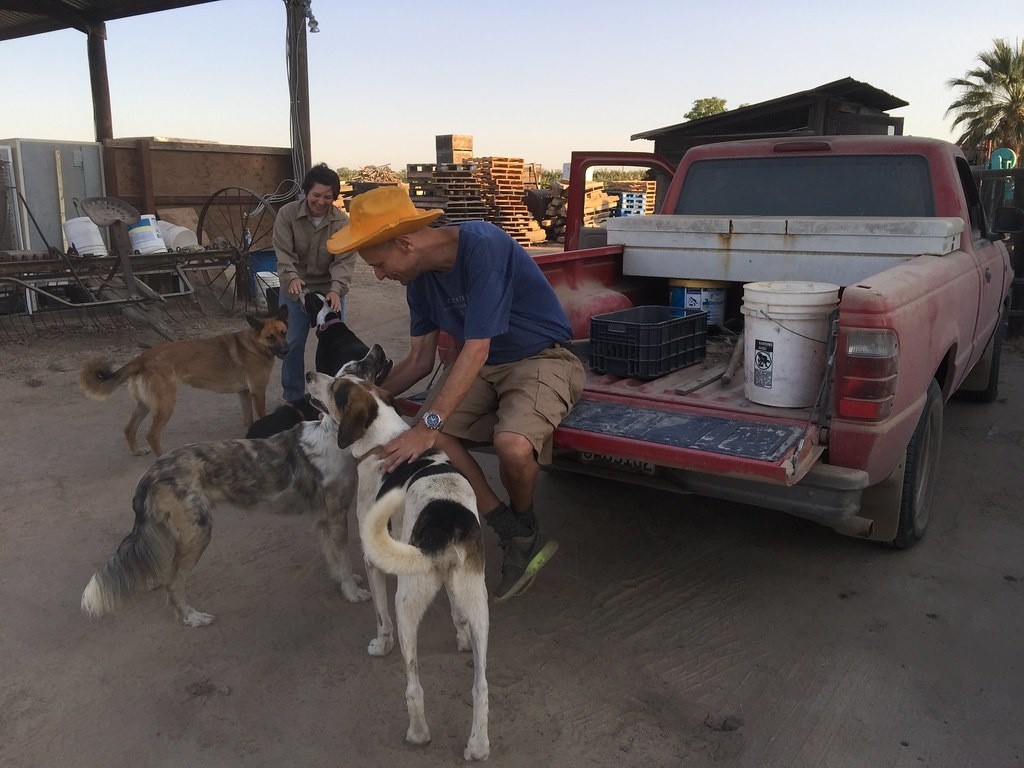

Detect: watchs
left=422, top=412, right=445, bottom=432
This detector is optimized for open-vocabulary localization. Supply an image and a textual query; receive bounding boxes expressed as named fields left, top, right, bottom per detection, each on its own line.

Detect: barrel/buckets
left=254, top=271, right=280, bottom=315
left=62, top=217, right=108, bottom=260
left=248, top=249, right=281, bottom=296
left=738, top=275, right=841, bottom=411
left=126, top=211, right=167, bottom=256
left=662, top=275, right=731, bottom=329
left=266, top=286, right=281, bottom=313
left=156, top=220, right=197, bottom=253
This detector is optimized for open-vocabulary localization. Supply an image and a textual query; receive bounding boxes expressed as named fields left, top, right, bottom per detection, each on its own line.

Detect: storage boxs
left=587, top=299, right=714, bottom=385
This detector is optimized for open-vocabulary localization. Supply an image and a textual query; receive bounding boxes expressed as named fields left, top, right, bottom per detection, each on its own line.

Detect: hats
left=326, top=184, right=444, bottom=255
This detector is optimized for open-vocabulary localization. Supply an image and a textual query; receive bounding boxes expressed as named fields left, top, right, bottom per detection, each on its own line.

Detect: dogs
left=81, top=285, right=492, bottom=761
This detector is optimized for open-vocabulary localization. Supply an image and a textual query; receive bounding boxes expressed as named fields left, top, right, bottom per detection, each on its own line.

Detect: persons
left=271, top=163, right=357, bottom=404
left=325, top=184, right=588, bottom=602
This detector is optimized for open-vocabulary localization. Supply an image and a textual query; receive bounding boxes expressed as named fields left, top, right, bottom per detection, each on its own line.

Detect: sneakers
left=493, top=510, right=559, bottom=605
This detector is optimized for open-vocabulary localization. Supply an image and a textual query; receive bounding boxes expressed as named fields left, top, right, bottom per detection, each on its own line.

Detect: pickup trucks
left=384, top=132, right=1015, bottom=554
